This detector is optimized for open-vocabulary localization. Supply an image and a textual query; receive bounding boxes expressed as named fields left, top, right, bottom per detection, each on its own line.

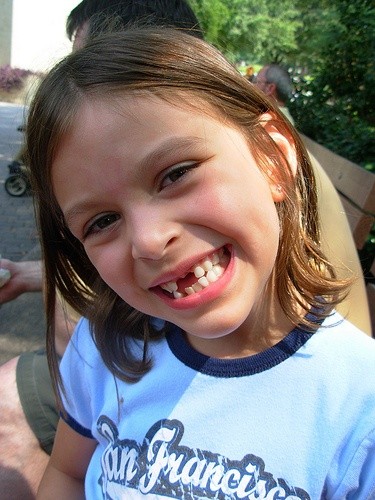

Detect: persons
left=1, top=0, right=374, bottom=500
left=228, top=50, right=315, bottom=138
left=20, top=26, right=375, bottom=499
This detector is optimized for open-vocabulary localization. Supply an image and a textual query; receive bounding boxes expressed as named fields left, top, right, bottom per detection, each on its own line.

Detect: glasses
left=252, top=78, right=272, bottom=87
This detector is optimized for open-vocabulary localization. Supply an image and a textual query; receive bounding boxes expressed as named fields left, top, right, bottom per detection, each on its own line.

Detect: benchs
left=299, top=133, right=375, bottom=250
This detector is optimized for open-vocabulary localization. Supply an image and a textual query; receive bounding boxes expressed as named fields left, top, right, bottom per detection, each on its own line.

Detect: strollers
left=4, top=126, right=33, bottom=198
